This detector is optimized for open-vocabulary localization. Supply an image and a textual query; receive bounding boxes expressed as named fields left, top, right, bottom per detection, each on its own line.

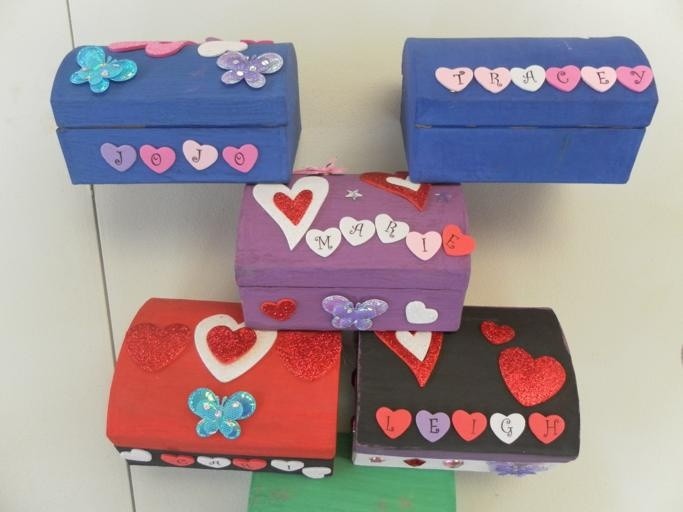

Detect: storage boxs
left=400, top=37, right=658, bottom=184
left=107, top=296, right=348, bottom=477
left=50, top=38, right=303, bottom=184
left=234, top=173, right=472, bottom=333
left=349, top=306, right=580, bottom=472
